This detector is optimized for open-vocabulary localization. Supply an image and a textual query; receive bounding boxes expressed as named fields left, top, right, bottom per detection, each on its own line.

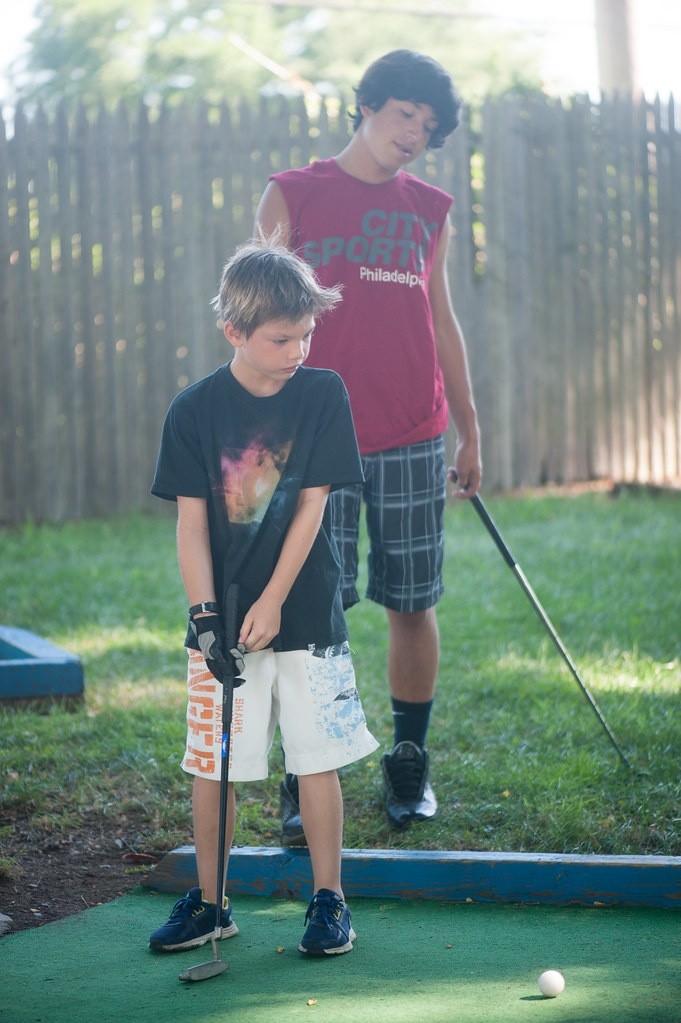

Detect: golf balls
left=539, top=968, right=564, bottom=997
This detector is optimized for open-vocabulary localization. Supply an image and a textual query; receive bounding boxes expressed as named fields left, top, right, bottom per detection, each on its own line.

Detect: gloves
left=193, top=616, right=246, bottom=688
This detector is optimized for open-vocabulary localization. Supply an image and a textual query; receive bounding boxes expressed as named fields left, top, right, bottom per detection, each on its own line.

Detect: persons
left=148, top=221, right=382, bottom=955
left=251, top=49, right=483, bottom=846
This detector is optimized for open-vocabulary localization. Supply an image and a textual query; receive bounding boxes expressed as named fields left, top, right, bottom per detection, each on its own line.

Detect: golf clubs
left=453, top=471, right=636, bottom=769
left=184, top=586, right=242, bottom=982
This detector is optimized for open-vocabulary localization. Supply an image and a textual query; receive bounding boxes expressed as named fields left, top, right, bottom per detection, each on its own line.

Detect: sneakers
left=297, top=888, right=356, bottom=954
left=148, top=888, right=238, bottom=951
left=380, top=741, right=438, bottom=830
left=279, top=778, right=307, bottom=846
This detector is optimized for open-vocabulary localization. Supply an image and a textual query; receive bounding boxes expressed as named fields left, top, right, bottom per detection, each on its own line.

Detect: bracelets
left=189, top=601, right=220, bottom=619
